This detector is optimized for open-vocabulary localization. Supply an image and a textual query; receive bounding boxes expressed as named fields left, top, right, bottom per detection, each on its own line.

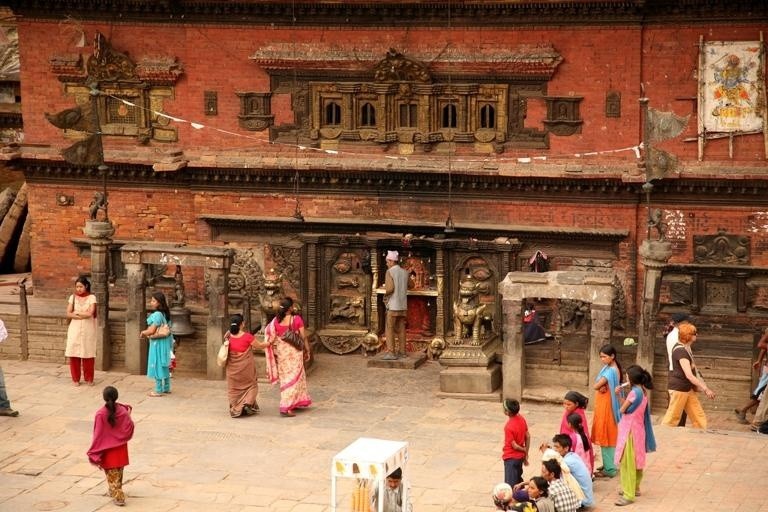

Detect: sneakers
left=619, top=490, right=641, bottom=497
left=615, top=496, right=633, bottom=505
left=0, top=408, right=19, bottom=416
left=594, top=471, right=611, bottom=477
left=382, top=354, right=397, bottom=360
left=280, top=410, right=296, bottom=417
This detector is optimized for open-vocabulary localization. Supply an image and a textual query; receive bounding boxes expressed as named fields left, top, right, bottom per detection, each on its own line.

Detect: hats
left=493, top=483, right=514, bottom=503
left=387, top=250, right=399, bottom=262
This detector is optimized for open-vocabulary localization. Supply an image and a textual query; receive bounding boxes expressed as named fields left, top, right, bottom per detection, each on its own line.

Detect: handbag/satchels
left=281, top=316, right=305, bottom=349
left=217, top=341, right=229, bottom=366
left=693, top=367, right=707, bottom=393
left=147, top=325, right=171, bottom=339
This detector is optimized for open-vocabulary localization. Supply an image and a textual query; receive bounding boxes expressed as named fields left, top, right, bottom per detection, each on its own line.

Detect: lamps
left=443, top=133, right=458, bottom=234
left=289, top=137, right=305, bottom=224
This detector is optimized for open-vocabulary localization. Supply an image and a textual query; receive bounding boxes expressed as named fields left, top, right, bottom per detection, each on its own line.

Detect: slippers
left=734, top=408, right=748, bottom=424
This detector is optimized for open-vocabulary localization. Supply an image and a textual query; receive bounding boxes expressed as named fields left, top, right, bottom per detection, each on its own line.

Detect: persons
left=491, top=391, right=595, bottom=512
left=614, top=365, right=656, bottom=507
left=0, top=319, right=19, bottom=417
left=224, top=314, right=275, bottom=418
left=139, top=292, right=174, bottom=397
left=709, top=54, right=757, bottom=118
left=663, top=311, right=768, bottom=435
left=63, top=275, right=99, bottom=387
left=87, top=385, right=135, bottom=506
left=590, top=343, right=625, bottom=478
left=525, top=308, right=553, bottom=345
left=265, top=296, right=311, bottom=417
left=371, top=467, right=413, bottom=512
left=383, top=250, right=415, bottom=361
